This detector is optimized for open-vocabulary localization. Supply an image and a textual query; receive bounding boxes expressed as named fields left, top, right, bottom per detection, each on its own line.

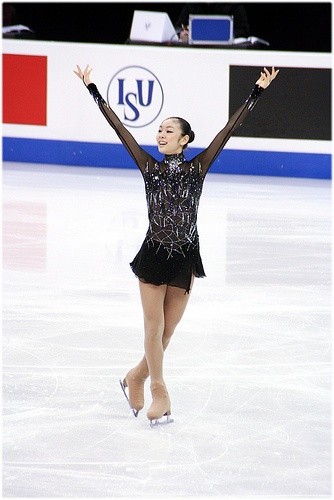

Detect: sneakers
left=119, top=361, right=149, bottom=417
left=146, top=381, right=174, bottom=426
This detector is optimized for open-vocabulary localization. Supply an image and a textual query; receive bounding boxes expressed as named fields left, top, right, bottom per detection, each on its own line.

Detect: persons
left=73, top=64, right=280, bottom=427
